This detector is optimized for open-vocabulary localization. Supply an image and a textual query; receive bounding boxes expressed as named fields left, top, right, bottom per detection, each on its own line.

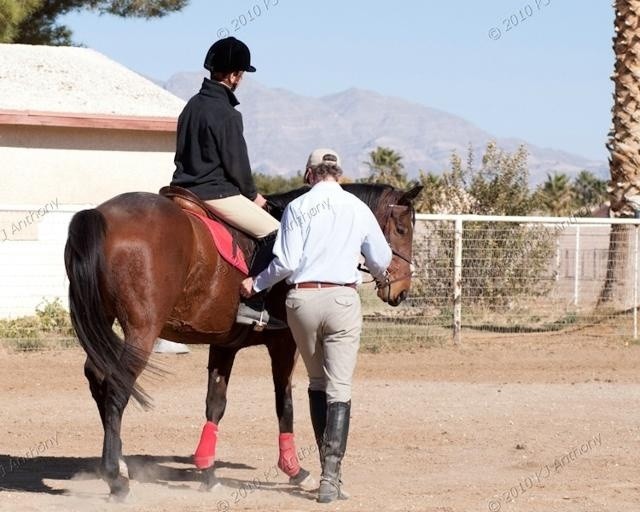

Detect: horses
left=64, top=180, right=425, bottom=504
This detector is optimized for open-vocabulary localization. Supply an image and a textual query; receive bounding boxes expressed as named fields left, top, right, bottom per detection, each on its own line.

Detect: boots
left=306, top=387, right=351, bottom=503
left=235, top=226, right=289, bottom=331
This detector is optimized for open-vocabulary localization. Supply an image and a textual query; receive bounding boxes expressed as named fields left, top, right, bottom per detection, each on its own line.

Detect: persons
left=168, top=36, right=290, bottom=331
left=239, top=148, right=394, bottom=504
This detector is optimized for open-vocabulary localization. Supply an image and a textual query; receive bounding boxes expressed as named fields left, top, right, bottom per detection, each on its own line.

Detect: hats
left=203, top=36, right=256, bottom=73
left=303, top=147, right=341, bottom=185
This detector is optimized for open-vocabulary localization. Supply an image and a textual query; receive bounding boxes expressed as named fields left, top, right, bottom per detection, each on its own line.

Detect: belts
left=290, top=280, right=357, bottom=291
